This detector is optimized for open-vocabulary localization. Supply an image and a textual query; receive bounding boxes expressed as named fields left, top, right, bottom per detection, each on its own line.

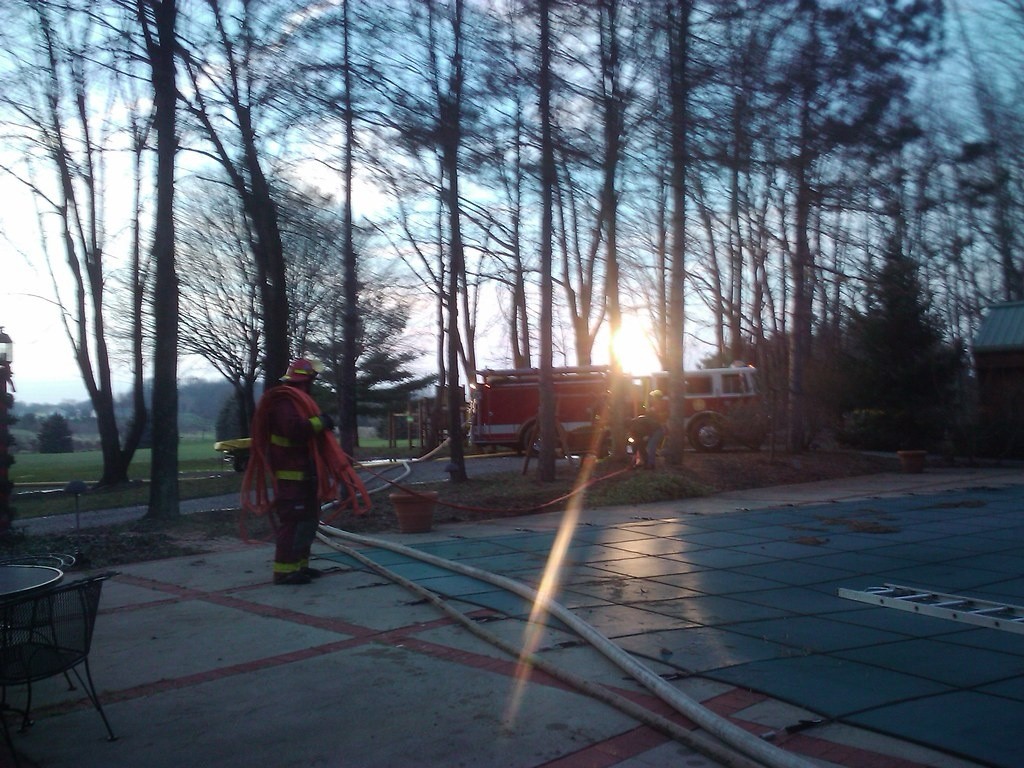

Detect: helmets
left=278, top=359, right=317, bottom=383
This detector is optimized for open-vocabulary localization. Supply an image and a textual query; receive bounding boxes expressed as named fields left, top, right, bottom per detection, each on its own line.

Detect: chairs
left=0, top=571, right=122, bottom=768
left=0, top=553, right=78, bottom=725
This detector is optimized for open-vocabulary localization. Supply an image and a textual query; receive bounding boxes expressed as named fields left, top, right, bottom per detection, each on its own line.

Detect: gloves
left=319, top=414, right=335, bottom=430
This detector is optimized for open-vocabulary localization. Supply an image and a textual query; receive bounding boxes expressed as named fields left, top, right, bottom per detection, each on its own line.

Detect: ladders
left=839, top=583, right=1024, bottom=636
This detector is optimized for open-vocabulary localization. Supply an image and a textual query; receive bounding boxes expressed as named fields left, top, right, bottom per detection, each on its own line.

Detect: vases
left=897, top=450, right=928, bottom=473
left=388, top=491, right=439, bottom=534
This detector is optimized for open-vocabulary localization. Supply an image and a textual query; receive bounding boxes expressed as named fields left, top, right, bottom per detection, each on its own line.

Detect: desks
left=0, top=564, right=64, bottom=606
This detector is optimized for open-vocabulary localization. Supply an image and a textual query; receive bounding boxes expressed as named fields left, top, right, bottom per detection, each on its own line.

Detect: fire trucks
left=468, top=362, right=773, bottom=455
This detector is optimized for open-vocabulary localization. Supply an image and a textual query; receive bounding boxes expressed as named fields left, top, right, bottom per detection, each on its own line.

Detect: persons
left=624, top=415, right=663, bottom=469
left=266, top=359, right=335, bottom=586
left=648, top=388, right=673, bottom=456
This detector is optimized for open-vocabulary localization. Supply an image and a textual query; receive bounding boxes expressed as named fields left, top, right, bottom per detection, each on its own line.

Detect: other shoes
left=273, top=570, right=313, bottom=584
left=301, top=565, right=322, bottom=578
